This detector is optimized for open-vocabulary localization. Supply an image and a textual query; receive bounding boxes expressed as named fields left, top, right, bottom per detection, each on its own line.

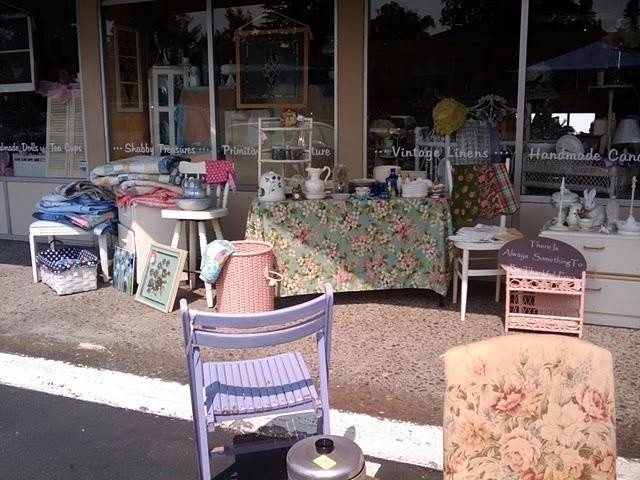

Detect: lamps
left=612, top=119, right=640, bottom=161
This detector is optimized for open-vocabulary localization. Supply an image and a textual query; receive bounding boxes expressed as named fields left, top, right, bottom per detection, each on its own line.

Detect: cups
left=355, top=187, right=371, bottom=196
left=272, top=145, right=285, bottom=160
left=290, top=147, right=302, bottom=160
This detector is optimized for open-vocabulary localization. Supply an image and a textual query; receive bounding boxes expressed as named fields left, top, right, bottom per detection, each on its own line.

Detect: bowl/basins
left=351, top=179, right=375, bottom=192
left=175, top=197, right=211, bottom=211
left=579, top=219, right=593, bottom=228
left=374, top=166, right=401, bottom=183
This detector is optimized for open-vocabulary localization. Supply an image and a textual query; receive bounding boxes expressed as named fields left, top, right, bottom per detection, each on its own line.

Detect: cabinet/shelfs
left=257, top=117, right=312, bottom=188
left=538, top=224, right=639, bottom=329
left=504, top=267, right=586, bottom=339
left=0, top=13, right=36, bottom=93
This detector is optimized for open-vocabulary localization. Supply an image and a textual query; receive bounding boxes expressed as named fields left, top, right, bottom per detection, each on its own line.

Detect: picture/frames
left=236, top=29, right=309, bottom=108
left=134, top=242, right=188, bottom=315
left=114, top=25, right=142, bottom=111
left=112, top=245, right=135, bottom=296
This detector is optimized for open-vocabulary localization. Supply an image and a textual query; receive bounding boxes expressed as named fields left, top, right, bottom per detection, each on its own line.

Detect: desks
left=255, top=190, right=447, bottom=301
left=29, top=217, right=118, bottom=283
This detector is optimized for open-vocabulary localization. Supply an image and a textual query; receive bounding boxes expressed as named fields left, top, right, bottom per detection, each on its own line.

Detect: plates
left=402, top=185, right=428, bottom=198
left=303, top=192, right=326, bottom=199
left=331, top=193, right=350, bottom=200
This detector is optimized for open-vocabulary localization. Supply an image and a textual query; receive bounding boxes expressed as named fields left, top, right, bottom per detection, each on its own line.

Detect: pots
left=285, top=433, right=367, bottom=480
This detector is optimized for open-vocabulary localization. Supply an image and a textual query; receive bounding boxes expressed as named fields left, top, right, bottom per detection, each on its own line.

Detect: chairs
left=161, top=162, right=233, bottom=307
left=445, top=158, right=524, bottom=321
left=441, top=335, right=616, bottom=480
left=179, top=284, right=334, bottom=479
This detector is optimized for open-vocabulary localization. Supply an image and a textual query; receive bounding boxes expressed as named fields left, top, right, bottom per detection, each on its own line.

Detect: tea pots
left=181, top=176, right=204, bottom=199
left=305, top=165, right=331, bottom=193
